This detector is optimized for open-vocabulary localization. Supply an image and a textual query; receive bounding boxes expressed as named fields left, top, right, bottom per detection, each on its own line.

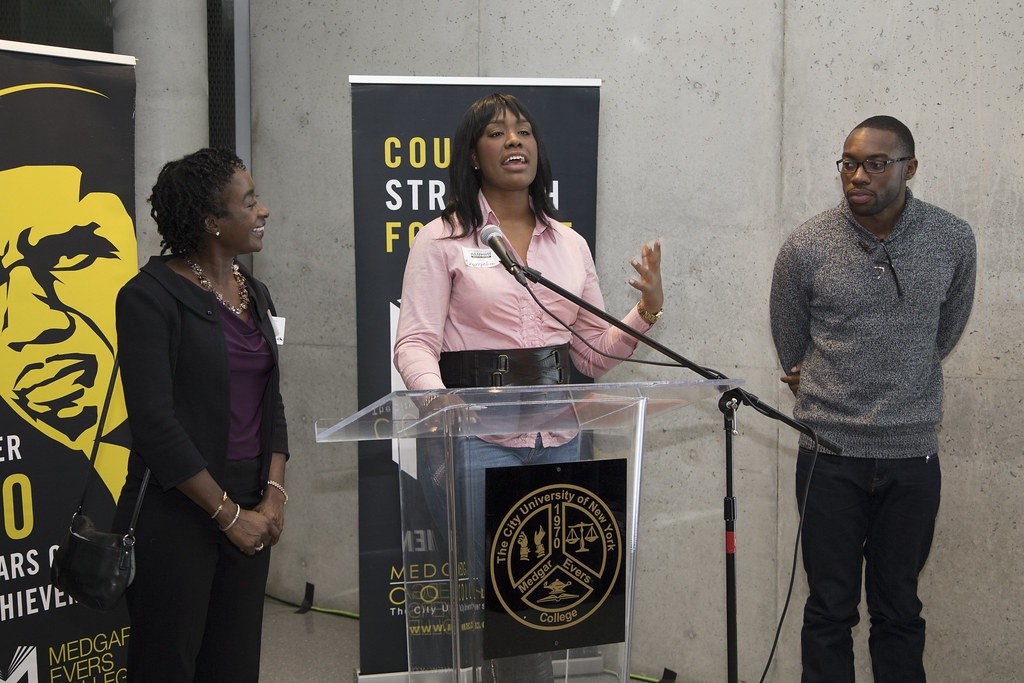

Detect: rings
left=256, top=544, right=263, bottom=550
left=430, top=408, right=441, bottom=414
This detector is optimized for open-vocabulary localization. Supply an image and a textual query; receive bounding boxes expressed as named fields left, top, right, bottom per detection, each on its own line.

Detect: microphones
left=479, top=225, right=527, bottom=286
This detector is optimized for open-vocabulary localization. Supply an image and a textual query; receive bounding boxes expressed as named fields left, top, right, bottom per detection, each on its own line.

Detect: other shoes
left=481, top=651, right=554, bottom=683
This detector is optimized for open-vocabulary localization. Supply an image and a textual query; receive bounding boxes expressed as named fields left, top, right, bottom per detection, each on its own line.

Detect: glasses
left=836, top=157, right=911, bottom=174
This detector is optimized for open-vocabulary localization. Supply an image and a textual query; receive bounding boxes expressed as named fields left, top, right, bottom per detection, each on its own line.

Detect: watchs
left=637, top=298, right=665, bottom=324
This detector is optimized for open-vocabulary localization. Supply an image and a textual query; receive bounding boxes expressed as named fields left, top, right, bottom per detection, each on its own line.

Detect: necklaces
left=179, top=246, right=248, bottom=315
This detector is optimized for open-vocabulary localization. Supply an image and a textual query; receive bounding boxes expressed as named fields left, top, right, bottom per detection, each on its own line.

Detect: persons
left=403, top=94, right=665, bottom=683
left=114, top=147, right=290, bottom=683
left=771, top=116, right=975, bottom=683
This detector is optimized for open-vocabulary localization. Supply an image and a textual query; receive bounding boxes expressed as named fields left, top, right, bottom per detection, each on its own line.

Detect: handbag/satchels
left=50, top=516, right=136, bottom=611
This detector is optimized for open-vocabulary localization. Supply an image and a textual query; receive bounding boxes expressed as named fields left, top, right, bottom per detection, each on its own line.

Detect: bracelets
left=421, top=393, right=440, bottom=408
left=219, top=504, right=240, bottom=530
left=267, top=481, right=288, bottom=504
left=211, top=491, right=226, bottom=519
